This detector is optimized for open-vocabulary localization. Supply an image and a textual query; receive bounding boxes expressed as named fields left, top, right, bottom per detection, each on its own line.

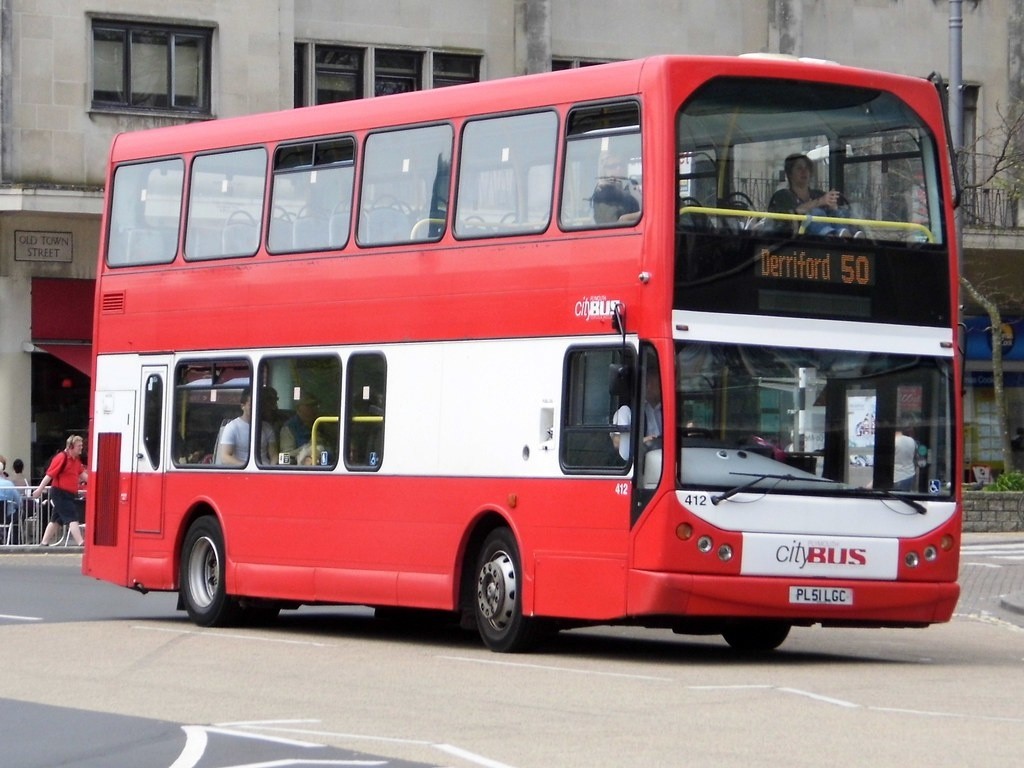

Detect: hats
left=259, top=388, right=279, bottom=401
left=293, top=392, right=322, bottom=406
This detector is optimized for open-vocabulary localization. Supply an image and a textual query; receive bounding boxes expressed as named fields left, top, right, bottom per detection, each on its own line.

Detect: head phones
left=69, top=436, right=75, bottom=450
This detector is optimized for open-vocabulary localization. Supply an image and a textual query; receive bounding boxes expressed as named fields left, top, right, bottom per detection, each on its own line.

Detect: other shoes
left=39, top=543, right=49, bottom=546
left=80, top=541, right=84, bottom=546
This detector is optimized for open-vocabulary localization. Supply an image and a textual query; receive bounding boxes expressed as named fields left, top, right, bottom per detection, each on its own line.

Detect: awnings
left=35, top=343, right=92, bottom=380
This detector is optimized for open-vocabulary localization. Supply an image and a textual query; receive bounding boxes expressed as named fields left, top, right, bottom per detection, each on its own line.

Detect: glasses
left=307, top=403, right=321, bottom=409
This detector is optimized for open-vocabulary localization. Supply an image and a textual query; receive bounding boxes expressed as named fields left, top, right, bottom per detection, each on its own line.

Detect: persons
left=892, top=417, right=917, bottom=492
left=766, top=153, right=868, bottom=238
left=0, top=456, right=29, bottom=523
left=594, top=151, right=634, bottom=181
left=214, top=387, right=335, bottom=469
left=33, top=435, right=88, bottom=547
left=608, top=368, right=664, bottom=461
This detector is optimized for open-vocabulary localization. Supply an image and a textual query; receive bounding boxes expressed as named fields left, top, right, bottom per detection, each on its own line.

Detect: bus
left=81, top=53, right=970, bottom=655
